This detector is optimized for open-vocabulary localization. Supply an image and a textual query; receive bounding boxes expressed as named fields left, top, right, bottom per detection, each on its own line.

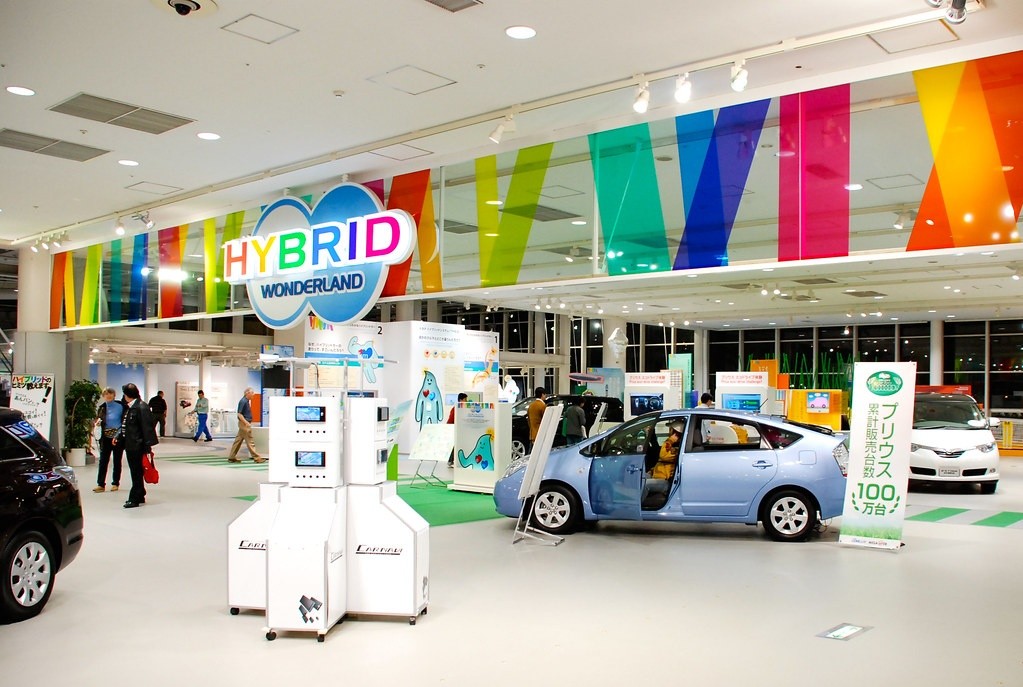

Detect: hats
left=666, top=421, right=684, bottom=433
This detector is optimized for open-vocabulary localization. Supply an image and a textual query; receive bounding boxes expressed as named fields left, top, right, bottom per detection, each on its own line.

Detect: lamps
left=674, top=70, right=693, bottom=103
left=945, top=0, right=967, bottom=24
left=924, top=0, right=943, bottom=8
left=535, top=297, right=604, bottom=315
left=761, top=283, right=817, bottom=303
left=844, top=326, right=849, bottom=334
left=463, top=298, right=499, bottom=312
left=633, top=82, right=650, bottom=113
left=846, top=308, right=882, bottom=318
left=489, top=114, right=514, bottom=144
left=30, top=230, right=67, bottom=253
left=131, top=211, right=156, bottom=230
left=658, top=314, right=689, bottom=327
left=730, top=59, right=748, bottom=92
left=564, top=250, right=579, bottom=263
left=114, top=217, right=125, bottom=236
left=893, top=209, right=920, bottom=230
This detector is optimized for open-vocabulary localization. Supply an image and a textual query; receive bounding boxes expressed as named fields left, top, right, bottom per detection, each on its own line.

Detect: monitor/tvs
left=377, top=407, right=389, bottom=422
left=295, top=451, right=325, bottom=468
left=722, top=394, right=761, bottom=414
left=296, top=406, right=325, bottom=422
left=377, top=448, right=387, bottom=463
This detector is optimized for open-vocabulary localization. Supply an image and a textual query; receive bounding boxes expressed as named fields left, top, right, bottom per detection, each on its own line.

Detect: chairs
left=646, top=429, right=704, bottom=504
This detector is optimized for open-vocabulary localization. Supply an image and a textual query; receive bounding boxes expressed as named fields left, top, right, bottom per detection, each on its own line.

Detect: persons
left=447, top=393, right=467, bottom=468
left=504, top=375, right=519, bottom=403
left=112, top=383, right=158, bottom=508
left=641, top=421, right=684, bottom=503
left=190, top=390, right=212, bottom=442
left=528, top=387, right=547, bottom=443
left=148, top=391, right=167, bottom=436
left=93, top=387, right=127, bottom=492
left=565, top=397, right=586, bottom=444
left=694, top=393, right=712, bottom=434
left=228, top=388, right=266, bottom=463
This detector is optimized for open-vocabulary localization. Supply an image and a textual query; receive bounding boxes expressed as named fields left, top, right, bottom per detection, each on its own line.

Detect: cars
left=0, top=407, right=88, bottom=621
left=909, top=392, right=1001, bottom=494
left=491, top=407, right=850, bottom=543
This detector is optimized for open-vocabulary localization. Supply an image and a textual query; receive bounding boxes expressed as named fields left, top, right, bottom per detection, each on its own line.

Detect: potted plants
left=64, top=379, right=102, bottom=467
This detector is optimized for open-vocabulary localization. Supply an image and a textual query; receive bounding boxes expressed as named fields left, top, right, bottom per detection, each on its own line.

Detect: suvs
left=512, top=395, right=626, bottom=464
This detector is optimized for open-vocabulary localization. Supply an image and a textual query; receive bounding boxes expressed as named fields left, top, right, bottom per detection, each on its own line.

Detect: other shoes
left=92, top=486, right=105, bottom=492
left=192, top=436, right=197, bottom=442
left=204, top=438, right=212, bottom=442
left=255, top=458, right=266, bottom=463
left=110, top=485, right=118, bottom=491
left=123, top=501, right=139, bottom=508
left=126, top=497, right=146, bottom=503
left=229, top=459, right=241, bottom=462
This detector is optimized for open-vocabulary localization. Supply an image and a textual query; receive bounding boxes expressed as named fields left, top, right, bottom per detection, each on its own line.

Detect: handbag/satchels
left=142, top=452, right=159, bottom=484
left=103, top=426, right=122, bottom=438
left=94, top=421, right=102, bottom=440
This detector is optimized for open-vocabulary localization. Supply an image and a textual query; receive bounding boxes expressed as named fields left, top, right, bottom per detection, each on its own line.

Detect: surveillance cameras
left=168, top=0, right=200, bottom=15
